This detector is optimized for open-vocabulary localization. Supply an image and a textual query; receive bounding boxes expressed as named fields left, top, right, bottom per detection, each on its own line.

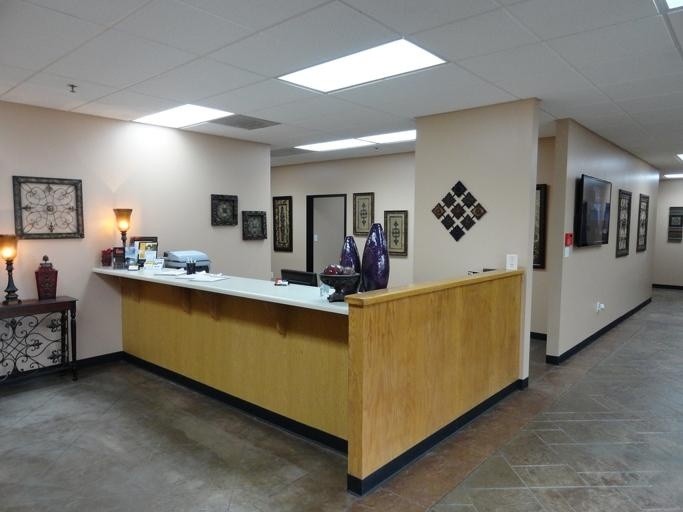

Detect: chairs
left=281, top=269, right=317, bottom=286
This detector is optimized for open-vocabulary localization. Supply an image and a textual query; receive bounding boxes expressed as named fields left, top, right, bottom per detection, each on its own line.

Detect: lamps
left=0, top=234, right=22, bottom=304
left=113, top=209, right=133, bottom=268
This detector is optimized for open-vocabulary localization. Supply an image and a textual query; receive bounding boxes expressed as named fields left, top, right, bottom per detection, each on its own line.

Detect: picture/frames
left=12, top=175, right=84, bottom=239
left=211, top=194, right=238, bottom=225
left=242, top=210, right=267, bottom=240
left=616, top=189, right=632, bottom=257
left=131, top=236, right=158, bottom=263
left=352, top=192, right=374, bottom=235
left=637, top=194, right=650, bottom=252
left=383, top=210, right=407, bottom=256
left=272, top=196, right=293, bottom=252
left=137, top=242, right=158, bottom=261
left=533, top=182, right=546, bottom=269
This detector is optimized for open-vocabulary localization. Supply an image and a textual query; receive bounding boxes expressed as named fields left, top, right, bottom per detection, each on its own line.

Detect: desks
left=0, top=296, right=79, bottom=381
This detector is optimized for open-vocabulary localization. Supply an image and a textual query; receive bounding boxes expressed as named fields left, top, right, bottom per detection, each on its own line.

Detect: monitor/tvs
left=573, top=173, right=612, bottom=247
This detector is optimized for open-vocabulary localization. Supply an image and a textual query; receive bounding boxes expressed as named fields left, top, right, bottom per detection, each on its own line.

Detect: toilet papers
left=506, top=254, right=518, bottom=271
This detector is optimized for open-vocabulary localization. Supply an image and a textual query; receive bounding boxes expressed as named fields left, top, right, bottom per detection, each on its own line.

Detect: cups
left=186, top=263, right=196, bottom=275
